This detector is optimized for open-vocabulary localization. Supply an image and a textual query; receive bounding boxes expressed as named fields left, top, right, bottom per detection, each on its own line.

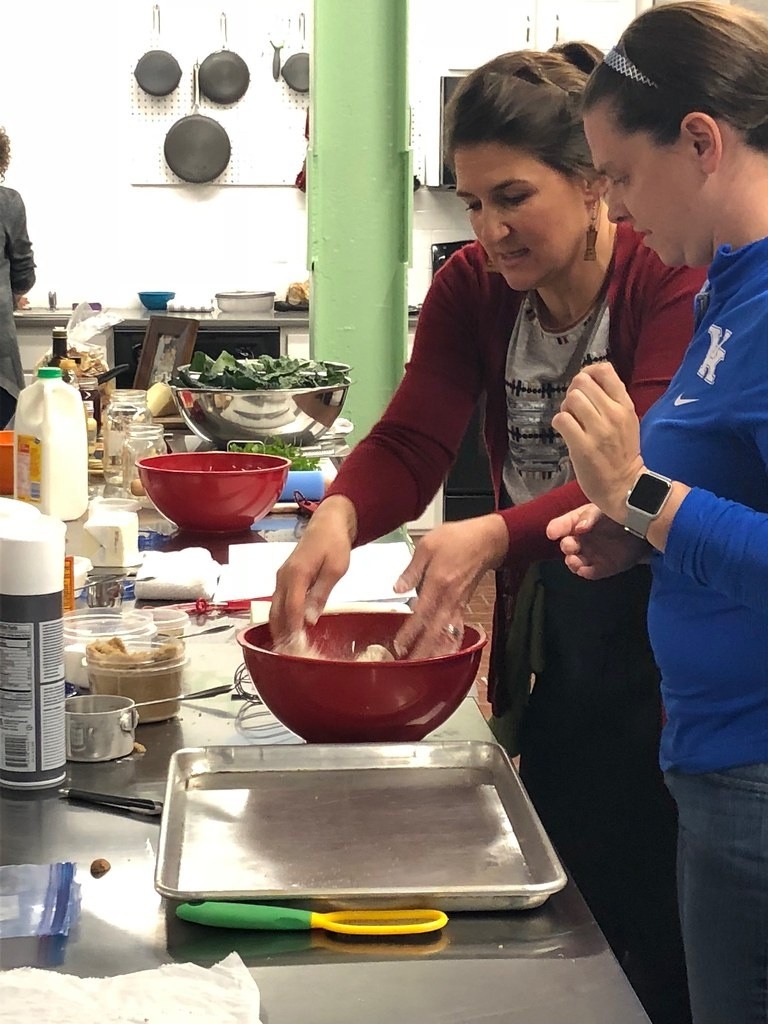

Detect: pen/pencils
left=57, top=787, right=163, bottom=815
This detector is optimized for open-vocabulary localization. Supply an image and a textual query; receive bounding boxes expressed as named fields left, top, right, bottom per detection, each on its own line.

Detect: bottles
left=12, top=366, right=88, bottom=521
left=102, top=388, right=157, bottom=487
left=48, top=326, right=69, bottom=367
left=122, top=423, right=168, bottom=499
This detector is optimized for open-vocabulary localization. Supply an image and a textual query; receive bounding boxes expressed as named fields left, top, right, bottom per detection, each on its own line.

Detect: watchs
left=624, top=470, right=674, bottom=539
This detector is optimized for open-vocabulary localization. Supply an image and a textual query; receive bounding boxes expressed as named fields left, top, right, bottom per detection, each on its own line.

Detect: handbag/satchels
left=505, top=561, right=545, bottom=690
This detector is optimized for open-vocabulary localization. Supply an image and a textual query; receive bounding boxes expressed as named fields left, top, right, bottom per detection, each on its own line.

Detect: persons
left=546, top=0, right=768, bottom=1024
left=268, top=43, right=704, bottom=1023
left=0, top=131, right=36, bottom=428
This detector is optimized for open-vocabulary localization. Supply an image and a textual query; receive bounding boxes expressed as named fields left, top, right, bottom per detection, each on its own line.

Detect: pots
left=133, top=4, right=309, bottom=183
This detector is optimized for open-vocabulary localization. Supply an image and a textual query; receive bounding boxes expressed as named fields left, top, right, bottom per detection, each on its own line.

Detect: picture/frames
left=132, top=315, right=200, bottom=390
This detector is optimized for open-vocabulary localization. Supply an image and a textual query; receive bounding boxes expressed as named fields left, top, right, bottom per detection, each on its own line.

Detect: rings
left=448, top=624, right=460, bottom=636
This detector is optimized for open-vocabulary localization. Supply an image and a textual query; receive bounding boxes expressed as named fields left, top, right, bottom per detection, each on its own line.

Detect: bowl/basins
left=138, top=291, right=176, bottom=310
left=170, top=357, right=356, bottom=447
left=135, top=452, right=292, bottom=533
left=236, top=610, right=488, bottom=742
left=64, top=694, right=139, bottom=763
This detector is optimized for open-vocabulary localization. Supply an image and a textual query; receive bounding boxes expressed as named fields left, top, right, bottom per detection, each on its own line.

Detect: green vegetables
left=168, top=351, right=351, bottom=392
left=230, top=434, right=320, bottom=471
